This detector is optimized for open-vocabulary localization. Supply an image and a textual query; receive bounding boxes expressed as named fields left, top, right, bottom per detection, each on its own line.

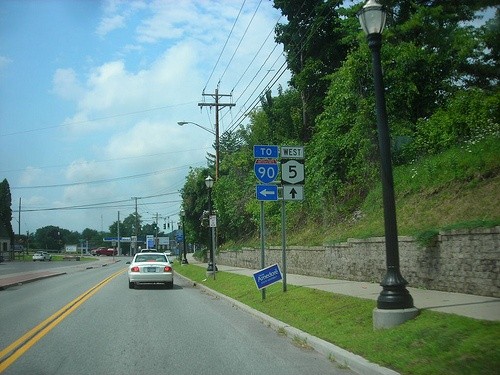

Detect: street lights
left=204, top=175, right=218, bottom=271
left=135, top=211, right=149, bottom=253
left=177, top=121, right=222, bottom=250
left=12, top=216, right=21, bottom=245
left=355, top=0, right=416, bottom=310
left=180, top=209, right=189, bottom=264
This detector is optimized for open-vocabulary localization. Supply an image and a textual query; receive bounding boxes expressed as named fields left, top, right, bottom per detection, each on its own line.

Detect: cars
left=126, top=252, right=174, bottom=289
left=134, top=249, right=158, bottom=259
left=163, top=250, right=173, bottom=256
left=33, top=251, right=52, bottom=262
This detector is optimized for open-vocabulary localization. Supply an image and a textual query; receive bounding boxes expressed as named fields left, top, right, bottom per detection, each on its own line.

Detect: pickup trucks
left=90, top=247, right=118, bottom=256
